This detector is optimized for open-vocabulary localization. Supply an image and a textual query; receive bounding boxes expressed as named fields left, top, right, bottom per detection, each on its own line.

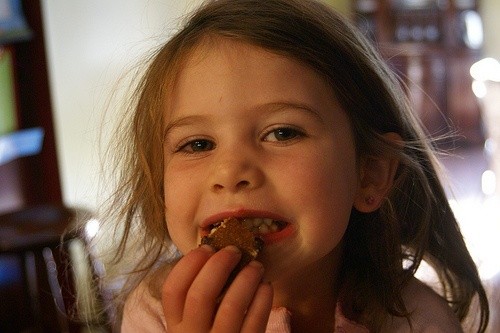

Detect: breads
left=198, top=216, right=262, bottom=277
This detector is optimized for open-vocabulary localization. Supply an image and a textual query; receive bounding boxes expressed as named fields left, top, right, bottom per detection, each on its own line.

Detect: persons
left=76, top=0, right=490, bottom=333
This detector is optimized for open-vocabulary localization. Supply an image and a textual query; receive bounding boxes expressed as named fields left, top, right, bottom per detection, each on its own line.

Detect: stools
left=1, top=205, right=113, bottom=333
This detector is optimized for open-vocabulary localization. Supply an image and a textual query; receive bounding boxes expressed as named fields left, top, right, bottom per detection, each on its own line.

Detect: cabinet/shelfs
left=1, top=2, right=66, bottom=314
left=357, top=4, right=484, bottom=150
